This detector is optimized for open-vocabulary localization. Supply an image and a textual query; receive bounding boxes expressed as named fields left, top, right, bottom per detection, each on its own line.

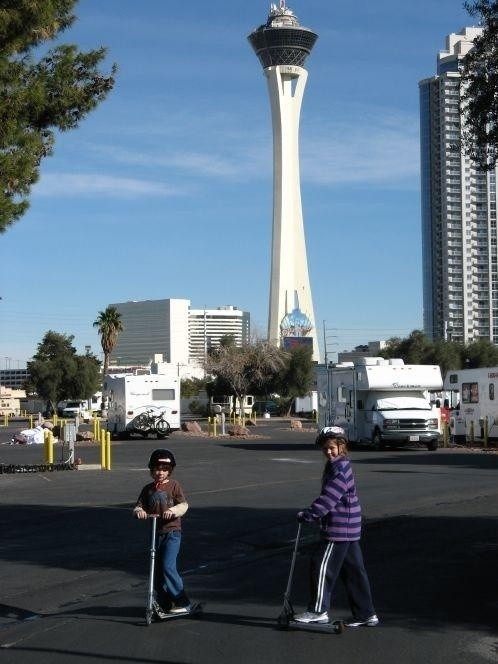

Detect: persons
left=131, top=449, right=194, bottom=614
left=292, top=425, right=381, bottom=627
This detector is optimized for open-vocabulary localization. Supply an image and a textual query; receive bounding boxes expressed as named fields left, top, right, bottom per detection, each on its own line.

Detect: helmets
left=316, top=426, right=348, bottom=443
left=148, top=448, right=175, bottom=469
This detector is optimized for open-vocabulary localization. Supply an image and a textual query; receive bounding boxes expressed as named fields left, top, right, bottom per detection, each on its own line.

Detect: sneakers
left=293, top=611, right=329, bottom=624
left=169, top=596, right=190, bottom=613
left=344, top=614, right=379, bottom=626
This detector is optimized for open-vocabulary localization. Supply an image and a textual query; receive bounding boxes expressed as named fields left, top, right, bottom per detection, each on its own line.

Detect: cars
left=63, top=400, right=90, bottom=418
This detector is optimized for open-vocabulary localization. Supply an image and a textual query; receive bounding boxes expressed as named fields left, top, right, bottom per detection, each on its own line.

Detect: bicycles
left=132, top=408, right=172, bottom=436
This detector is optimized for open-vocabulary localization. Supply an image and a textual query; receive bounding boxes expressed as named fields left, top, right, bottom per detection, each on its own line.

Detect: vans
left=315, top=357, right=447, bottom=454
left=442, top=365, right=498, bottom=447
left=105, top=372, right=183, bottom=440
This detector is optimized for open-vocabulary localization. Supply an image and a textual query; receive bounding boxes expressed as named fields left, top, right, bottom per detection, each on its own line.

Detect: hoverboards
left=136, top=506, right=203, bottom=627
left=275, top=511, right=345, bottom=634
